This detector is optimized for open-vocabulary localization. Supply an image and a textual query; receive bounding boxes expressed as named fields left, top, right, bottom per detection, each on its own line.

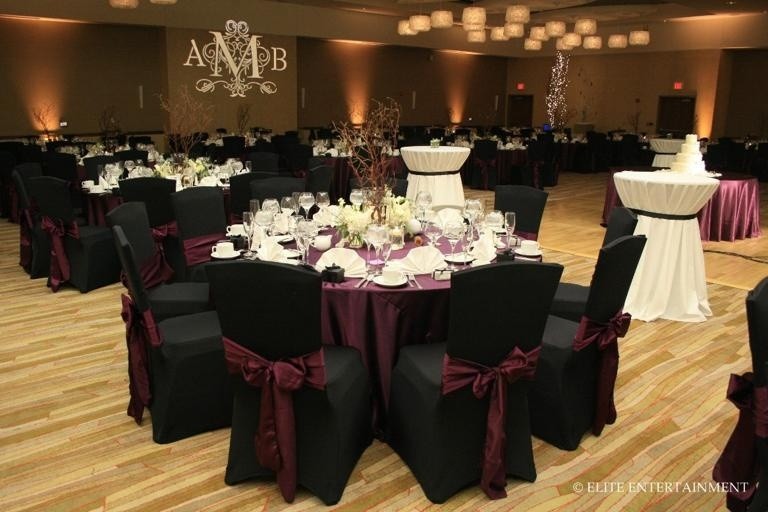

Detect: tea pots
left=310, top=235, right=333, bottom=251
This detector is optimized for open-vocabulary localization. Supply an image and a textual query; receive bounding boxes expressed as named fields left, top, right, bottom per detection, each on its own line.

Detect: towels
left=258, top=239, right=287, bottom=263
left=200, top=177, right=216, bottom=187
left=434, top=207, right=461, bottom=226
left=273, top=214, right=290, bottom=233
left=471, top=236, right=497, bottom=260
left=315, top=248, right=367, bottom=278
left=99, top=177, right=111, bottom=190
left=398, top=246, right=448, bottom=273
left=314, top=207, right=336, bottom=227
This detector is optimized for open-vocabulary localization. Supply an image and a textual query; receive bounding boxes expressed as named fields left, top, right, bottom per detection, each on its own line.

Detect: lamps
left=504, top=5, right=529, bottom=24
left=545, top=21, right=566, bottom=37
left=582, top=36, right=603, bottom=50
left=408, top=14, right=431, bottom=31
left=490, top=26, right=510, bottom=41
left=608, top=34, right=628, bottom=48
left=574, top=19, right=598, bottom=35
left=431, top=11, right=453, bottom=28
left=629, top=30, right=650, bottom=47
left=563, top=33, right=582, bottom=47
left=397, top=20, right=419, bottom=36
left=556, top=38, right=574, bottom=51
left=529, top=27, right=550, bottom=41
left=463, top=23, right=485, bottom=30
left=467, top=30, right=486, bottom=42
left=503, top=23, right=524, bottom=38
left=524, top=38, right=541, bottom=51
left=462, top=6, right=486, bottom=24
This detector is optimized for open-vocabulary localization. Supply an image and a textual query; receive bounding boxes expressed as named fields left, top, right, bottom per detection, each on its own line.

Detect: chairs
left=118, top=177, right=177, bottom=266
left=545, top=205, right=639, bottom=314
left=105, top=201, right=211, bottom=317
left=169, top=186, right=230, bottom=282
left=249, top=152, right=279, bottom=172
left=530, top=235, right=646, bottom=452
left=223, top=136, right=245, bottom=153
left=50, top=156, right=76, bottom=180
left=24, top=175, right=121, bottom=295
left=294, top=143, right=313, bottom=159
left=515, top=141, right=546, bottom=190
left=309, top=165, right=333, bottom=194
left=230, top=171, right=282, bottom=212
left=469, top=139, right=499, bottom=190
left=713, top=275, right=768, bottom=512
left=208, top=259, right=376, bottom=506
left=493, top=186, right=548, bottom=239
left=17, top=162, right=43, bottom=178
left=12, top=173, right=52, bottom=279
left=82, top=155, right=115, bottom=181
left=539, top=135, right=560, bottom=187
left=250, top=177, right=305, bottom=205
left=115, top=150, right=148, bottom=162
left=378, top=262, right=565, bottom=505
left=113, top=223, right=234, bottom=445
left=129, top=137, right=150, bottom=149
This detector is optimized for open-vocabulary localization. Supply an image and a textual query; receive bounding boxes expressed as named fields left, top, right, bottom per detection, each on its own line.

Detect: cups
left=226, top=225, right=243, bottom=233
left=256, top=210, right=272, bottom=247
left=389, top=227, right=403, bottom=251
left=212, top=243, right=233, bottom=256
left=521, top=240, right=538, bottom=253
left=250, top=200, right=260, bottom=220
left=382, top=266, right=402, bottom=282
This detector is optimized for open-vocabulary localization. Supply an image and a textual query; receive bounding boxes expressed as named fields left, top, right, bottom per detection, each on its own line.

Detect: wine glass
left=444, top=222, right=461, bottom=273
left=297, top=220, right=315, bottom=264
left=316, top=192, right=331, bottom=217
left=124, top=161, right=134, bottom=172
left=350, top=190, right=363, bottom=212
left=289, top=216, right=306, bottom=250
left=464, top=201, right=482, bottom=241
left=501, top=212, right=515, bottom=247
left=414, top=192, right=433, bottom=225
left=292, top=193, right=300, bottom=217
left=361, top=225, right=388, bottom=266
left=423, top=214, right=441, bottom=249
left=280, top=197, right=294, bottom=222
left=219, top=167, right=230, bottom=183
left=232, top=158, right=242, bottom=174
left=298, top=193, right=314, bottom=221
left=263, top=200, right=279, bottom=220
left=486, top=211, right=502, bottom=242
left=242, top=210, right=256, bottom=257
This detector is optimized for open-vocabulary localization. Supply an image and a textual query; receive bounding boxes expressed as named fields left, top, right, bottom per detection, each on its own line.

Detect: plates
left=392, top=255, right=447, bottom=275
left=373, top=274, right=408, bottom=286
left=444, top=254, right=474, bottom=263
left=258, top=248, right=300, bottom=259
left=211, top=252, right=241, bottom=259
left=516, top=248, right=542, bottom=257
left=224, top=231, right=243, bottom=238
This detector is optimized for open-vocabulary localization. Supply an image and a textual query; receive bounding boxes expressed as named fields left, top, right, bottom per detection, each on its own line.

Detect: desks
left=401, top=146, right=470, bottom=207
left=613, top=171, right=720, bottom=323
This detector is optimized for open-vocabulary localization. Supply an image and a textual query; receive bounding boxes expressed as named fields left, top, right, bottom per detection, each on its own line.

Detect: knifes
left=355, top=276, right=366, bottom=289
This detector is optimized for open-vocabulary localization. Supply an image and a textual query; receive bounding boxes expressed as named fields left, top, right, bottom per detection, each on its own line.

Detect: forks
left=407, top=272, right=422, bottom=289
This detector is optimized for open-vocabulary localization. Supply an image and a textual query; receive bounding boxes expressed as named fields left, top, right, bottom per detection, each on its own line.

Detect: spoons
left=363, top=274, right=373, bottom=289
left=403, top=272, right=414, bottom=288
